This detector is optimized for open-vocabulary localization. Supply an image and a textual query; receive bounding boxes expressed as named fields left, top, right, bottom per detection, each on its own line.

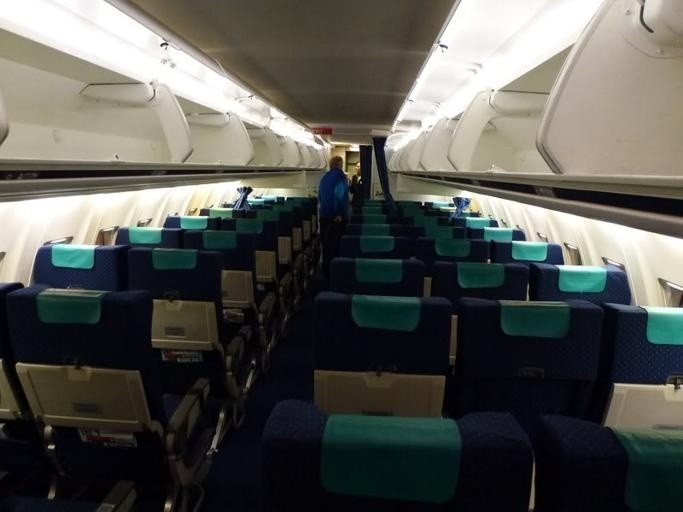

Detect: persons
left=347, top=161, right=364, bottom=207
left=318, top=155, right=350, bottom=260
